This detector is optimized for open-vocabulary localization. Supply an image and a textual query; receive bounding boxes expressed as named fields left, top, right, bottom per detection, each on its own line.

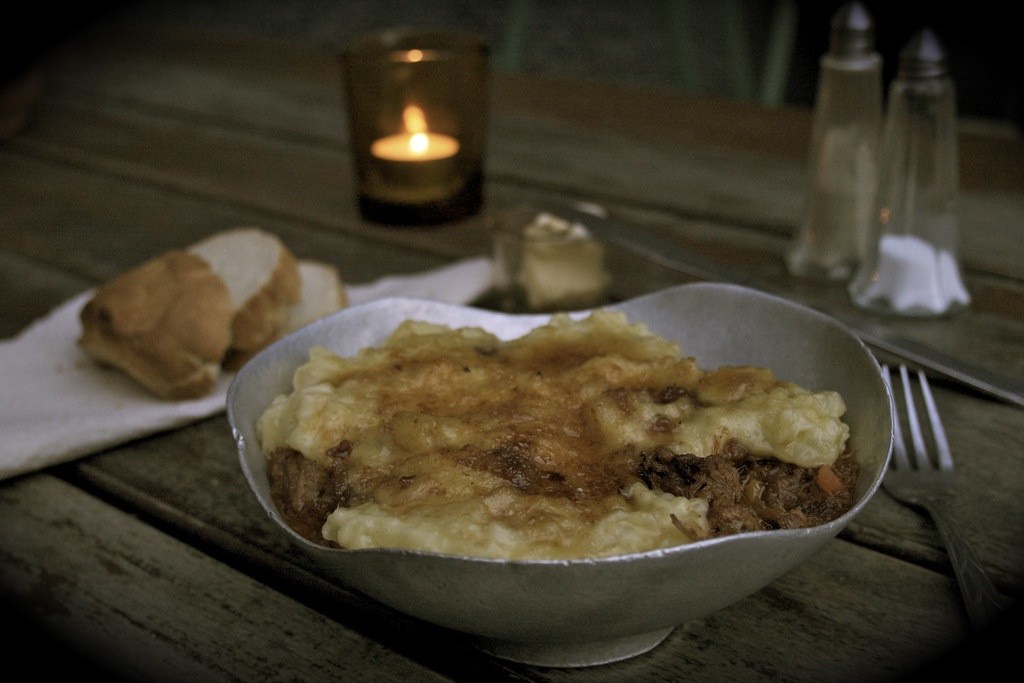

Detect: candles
left=371, top=131, right=461, bottom=190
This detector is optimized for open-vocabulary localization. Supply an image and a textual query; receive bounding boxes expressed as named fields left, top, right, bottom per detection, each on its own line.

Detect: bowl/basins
left=225, top=281, right=894, bottom=669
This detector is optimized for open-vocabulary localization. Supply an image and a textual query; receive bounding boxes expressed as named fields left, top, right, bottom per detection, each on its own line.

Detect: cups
left=484, top=207, right=625, bottom=315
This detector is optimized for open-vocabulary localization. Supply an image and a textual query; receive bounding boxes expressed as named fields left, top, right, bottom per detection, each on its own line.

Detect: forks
left=874, top=362, right=1024, bottom=683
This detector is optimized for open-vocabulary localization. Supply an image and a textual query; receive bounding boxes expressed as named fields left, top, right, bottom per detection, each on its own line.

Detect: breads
left=75, top=229, right=347, bottom=402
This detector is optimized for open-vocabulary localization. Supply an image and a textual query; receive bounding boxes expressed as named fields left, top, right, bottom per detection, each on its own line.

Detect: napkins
left=1, top=226, right=501, bottom=488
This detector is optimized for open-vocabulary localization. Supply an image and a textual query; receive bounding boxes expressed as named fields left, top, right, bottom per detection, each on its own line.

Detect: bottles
left=847, top=24, right=974, bottom=321
left=784, top=1, right=886, bottom=284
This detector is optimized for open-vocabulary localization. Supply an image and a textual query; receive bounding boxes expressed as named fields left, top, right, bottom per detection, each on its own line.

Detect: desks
left=1, top=27, right=1024, bottom=683
